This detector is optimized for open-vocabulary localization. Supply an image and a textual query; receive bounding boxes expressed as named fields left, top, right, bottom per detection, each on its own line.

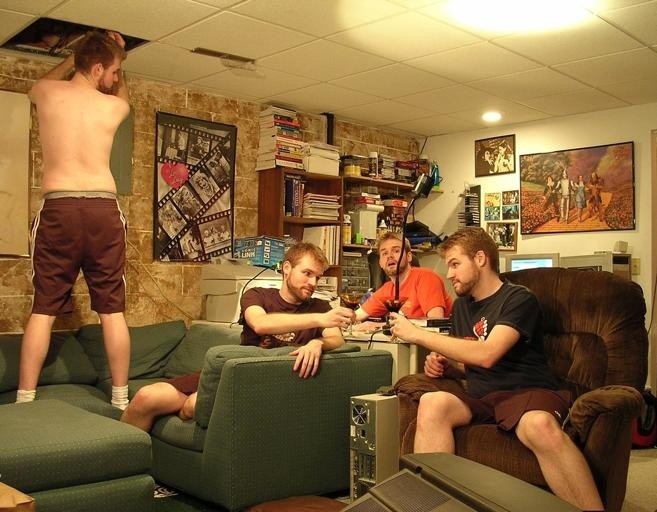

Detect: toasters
left=342, top=214, right=352, bottom=245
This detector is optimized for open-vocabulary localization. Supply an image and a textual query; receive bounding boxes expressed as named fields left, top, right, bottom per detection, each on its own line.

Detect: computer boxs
left=348, top=394, right=400, bottom=504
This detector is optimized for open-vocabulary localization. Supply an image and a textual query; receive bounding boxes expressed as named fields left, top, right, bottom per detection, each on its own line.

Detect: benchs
left=0, top=399, right=155, bottom=512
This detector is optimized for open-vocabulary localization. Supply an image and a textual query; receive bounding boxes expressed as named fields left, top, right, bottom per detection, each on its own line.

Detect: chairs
left=394, top=269, right=649, bottom=512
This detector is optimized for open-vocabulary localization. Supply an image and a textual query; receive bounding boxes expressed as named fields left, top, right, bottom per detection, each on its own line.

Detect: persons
left=388, top=226, right=604, bottom=510
left=476, top=143, right=513, bottom=174
left=159, top=131, right=229, bottom=258
left=490, top=225, right=514, bottom=248
left=120, top=243, right=355, bottom=432
left=16, top=32, right=130, bottom=409
left=355, top=233, right=452, bottom=322
left=543, top=170, right=604, bottom=224
left=486, top=192, right=519, bottom=219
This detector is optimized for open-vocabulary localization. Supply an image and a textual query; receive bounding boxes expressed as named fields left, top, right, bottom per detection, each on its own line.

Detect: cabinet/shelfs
left=343, top=173, right=443, bottom=305
left=459, top=185, right=481, bottom=227
left=257, top=168, right=343, bottom=303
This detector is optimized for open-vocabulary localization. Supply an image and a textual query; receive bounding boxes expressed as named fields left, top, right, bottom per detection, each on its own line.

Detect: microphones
left=411, top=174, right=436, bottom=199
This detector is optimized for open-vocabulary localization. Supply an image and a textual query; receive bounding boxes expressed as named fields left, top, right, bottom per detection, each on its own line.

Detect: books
left=255, top=103, right=406, bottom=266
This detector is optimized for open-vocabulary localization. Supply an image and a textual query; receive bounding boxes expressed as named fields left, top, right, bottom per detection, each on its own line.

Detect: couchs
left=0, top=320, right=393, bottom=512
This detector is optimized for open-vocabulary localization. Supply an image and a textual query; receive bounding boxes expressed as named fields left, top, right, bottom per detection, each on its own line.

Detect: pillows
left=0, top=326, right=100, bottom=394
left=78, top=322, right=187, bottom=391
left=167, top=321, right=246, bottom=380
left=194, top=345, right=360, bottom=438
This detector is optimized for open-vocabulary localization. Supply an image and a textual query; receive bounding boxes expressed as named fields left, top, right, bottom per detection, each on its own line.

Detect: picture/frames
left=519, top=141, right=636, bottom=235
left=486, top=222, right=517, bottom=251
left=475, top=134, right=516, bottom=177
left=155, top=110, right=237, bottom=263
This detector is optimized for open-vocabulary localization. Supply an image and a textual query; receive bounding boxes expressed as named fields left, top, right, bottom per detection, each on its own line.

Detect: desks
left=343, top=322, right=457, bottom=387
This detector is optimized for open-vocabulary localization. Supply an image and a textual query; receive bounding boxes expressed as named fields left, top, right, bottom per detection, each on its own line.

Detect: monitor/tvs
left=505, top=253, right=559, bottom=273
left=341, top=453, right=582, bottom=512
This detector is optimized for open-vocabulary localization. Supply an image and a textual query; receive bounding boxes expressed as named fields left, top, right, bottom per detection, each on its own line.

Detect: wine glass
left=377, top=296, right=409, bottom=343
left=339, top=290, right=363, bottom=339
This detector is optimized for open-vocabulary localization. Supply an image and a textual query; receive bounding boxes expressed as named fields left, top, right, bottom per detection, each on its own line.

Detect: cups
left=355, top=233, right=361, bottom=244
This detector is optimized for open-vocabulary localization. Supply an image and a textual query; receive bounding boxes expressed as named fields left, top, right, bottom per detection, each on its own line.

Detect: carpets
left=155, top=477, right=231, bottom=512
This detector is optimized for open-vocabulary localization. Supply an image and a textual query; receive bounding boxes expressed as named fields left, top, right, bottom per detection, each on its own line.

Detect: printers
left=200, top=263, right=340, bottom=323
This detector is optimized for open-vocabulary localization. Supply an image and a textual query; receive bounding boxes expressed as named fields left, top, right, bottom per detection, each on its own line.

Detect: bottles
left=343, top=158, right=361, bottom=177
left=363, top=237, right=368, bottom=245
left=431, top=160, right=440, bottom=189
left=369, top=152, right=378, bottom=176
